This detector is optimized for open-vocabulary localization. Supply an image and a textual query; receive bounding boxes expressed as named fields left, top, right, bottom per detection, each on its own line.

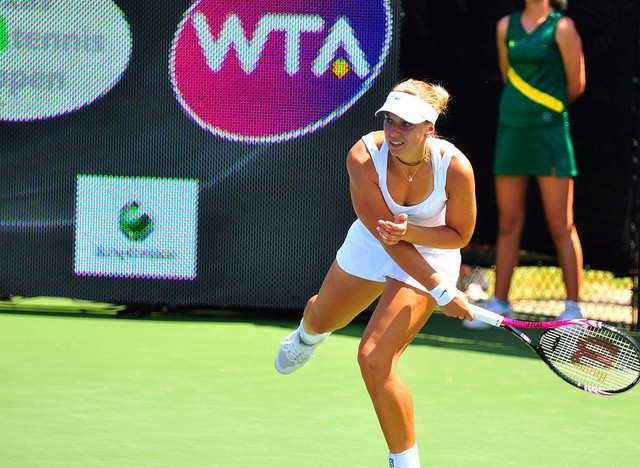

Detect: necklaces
left=387, top=150, right=431, bottom=184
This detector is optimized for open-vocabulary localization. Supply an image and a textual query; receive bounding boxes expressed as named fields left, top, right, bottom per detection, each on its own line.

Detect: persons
left=273, top=77, right=477, bottom=467
left=461, top=1, right=586, bottom=333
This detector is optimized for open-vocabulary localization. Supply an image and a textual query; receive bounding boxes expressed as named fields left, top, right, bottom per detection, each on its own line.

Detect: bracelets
left=425, top=279, right=460, bottom=308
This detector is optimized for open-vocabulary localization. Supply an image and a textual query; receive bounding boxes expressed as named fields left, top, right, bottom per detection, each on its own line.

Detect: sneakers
left=461, top=300, right=513, bottom=330
left=274, top=326, right=330, bottom=374
left=554, top=310, right=583, bottom=322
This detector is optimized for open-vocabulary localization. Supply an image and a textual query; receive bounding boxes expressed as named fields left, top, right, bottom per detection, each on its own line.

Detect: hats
left=374, top=90, right=438, bottom=125
